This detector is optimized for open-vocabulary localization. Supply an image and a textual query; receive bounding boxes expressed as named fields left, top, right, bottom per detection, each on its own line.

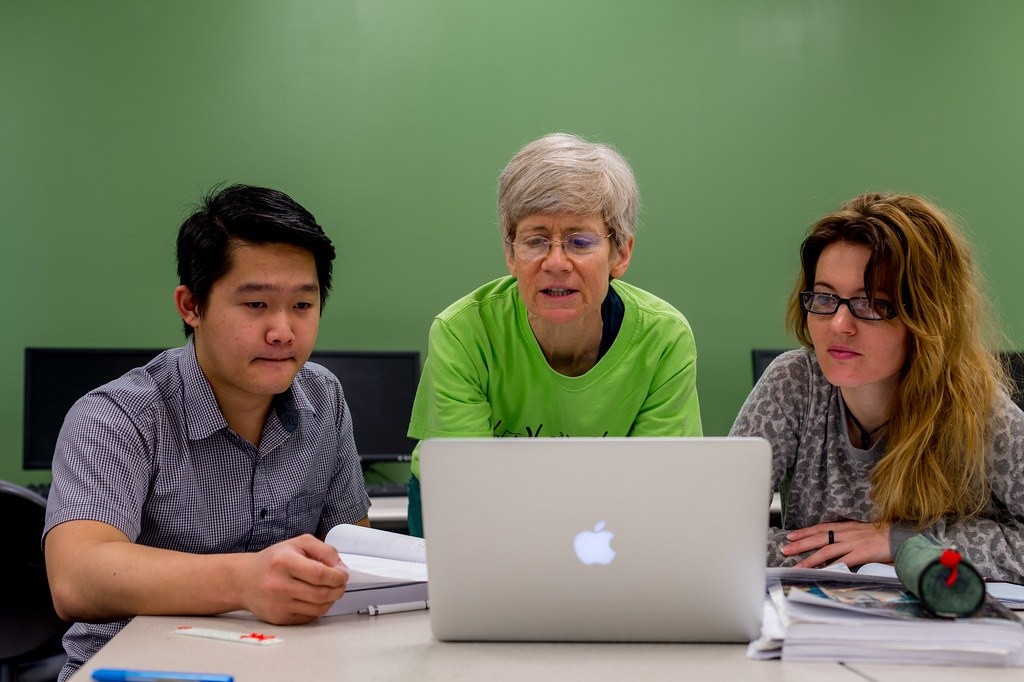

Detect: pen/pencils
left=90, top=668, right=233, bottom=682
left=357, top=599, right=431, bottom=616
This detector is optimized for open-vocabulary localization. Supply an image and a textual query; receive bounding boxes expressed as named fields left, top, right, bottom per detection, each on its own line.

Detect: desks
left=64, top=603, right=1024, bottom=682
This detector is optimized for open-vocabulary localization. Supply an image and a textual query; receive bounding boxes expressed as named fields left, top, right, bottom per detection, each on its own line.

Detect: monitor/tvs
left=752, top=348, right=785, bottom=384
left=307, top=350, right=421, bottom=460
left=22, top=347, right=174, bottom=470
left=995, top=352, right=1024, bottom=411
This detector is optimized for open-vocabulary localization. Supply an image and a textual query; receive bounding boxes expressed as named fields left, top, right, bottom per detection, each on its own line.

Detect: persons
left=726, top=191, right=1023, bottom=583
left=42, top=183, right=371, bottom=682
left=405, top=132, right=705, bottom=539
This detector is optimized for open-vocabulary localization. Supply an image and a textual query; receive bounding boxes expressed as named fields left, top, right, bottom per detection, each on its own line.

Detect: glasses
left=798, top=288, right=910, bottom=320
left=505, top=232, right=613, bottom=263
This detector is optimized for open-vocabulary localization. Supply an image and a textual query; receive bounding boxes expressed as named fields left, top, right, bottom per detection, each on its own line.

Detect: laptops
left=421, top=436, right=773, bottom=644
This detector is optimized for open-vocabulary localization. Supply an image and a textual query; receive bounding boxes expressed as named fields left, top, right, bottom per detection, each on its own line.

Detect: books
left=745, top=579, right=1024, bottom=665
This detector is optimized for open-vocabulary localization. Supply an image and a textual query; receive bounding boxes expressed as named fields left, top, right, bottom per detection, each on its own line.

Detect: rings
left=829, top=530, right=834, bottom=544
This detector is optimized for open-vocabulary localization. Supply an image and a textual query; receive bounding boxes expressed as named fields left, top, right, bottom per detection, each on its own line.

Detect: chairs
left=0, top=479, right=68, bottom=682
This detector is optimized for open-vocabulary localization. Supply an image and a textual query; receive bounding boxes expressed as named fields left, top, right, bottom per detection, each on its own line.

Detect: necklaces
left=844, top=403, right=888, bottom=450
